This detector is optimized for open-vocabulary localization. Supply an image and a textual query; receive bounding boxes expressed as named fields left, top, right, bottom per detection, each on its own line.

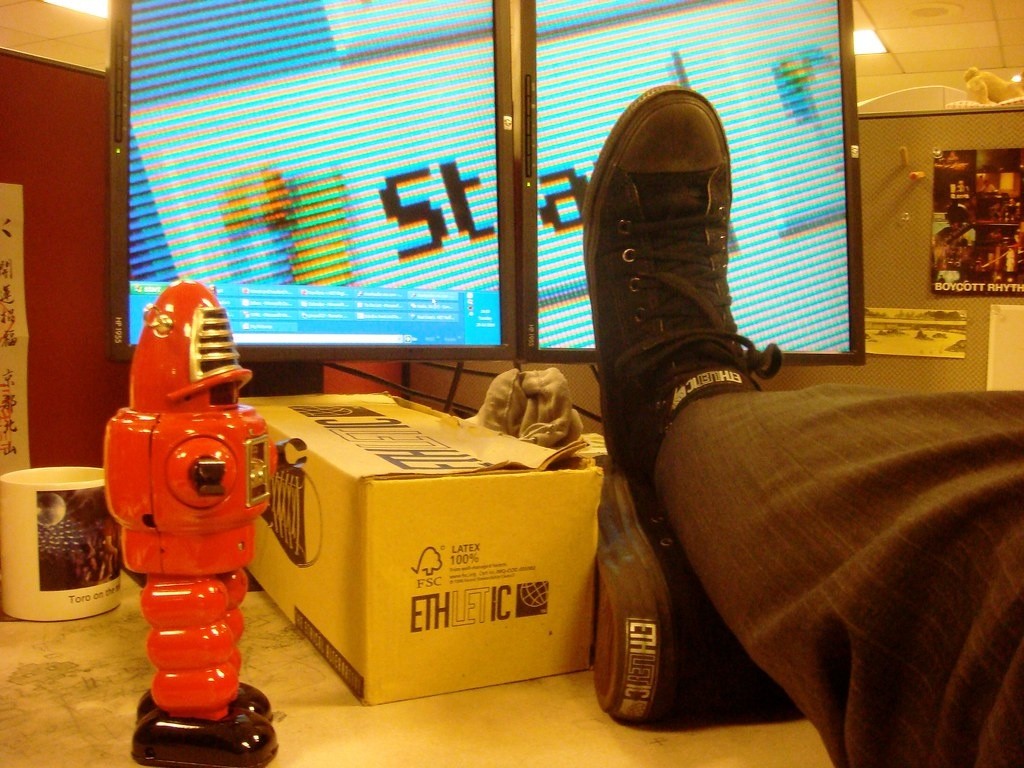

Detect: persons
left=583, top=86, right=1024, bottom=768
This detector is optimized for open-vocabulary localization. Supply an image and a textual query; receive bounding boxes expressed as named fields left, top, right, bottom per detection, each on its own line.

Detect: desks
left=0, top=569, right=839, bottom=768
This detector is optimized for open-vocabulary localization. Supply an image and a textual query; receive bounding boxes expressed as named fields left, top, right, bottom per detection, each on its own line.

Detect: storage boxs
left=239, top=390, right=604, bottom=707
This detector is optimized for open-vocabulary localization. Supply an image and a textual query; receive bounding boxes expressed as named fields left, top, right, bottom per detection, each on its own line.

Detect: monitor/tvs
left=107, top=0, right=520, bottom=396
left=518, top=0, right=868, bottom=365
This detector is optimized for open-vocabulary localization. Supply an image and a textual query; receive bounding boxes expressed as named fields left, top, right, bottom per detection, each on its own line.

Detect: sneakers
left=595, top=450, right=770, bottom=725
left=583, top=85, right=780, bottom=495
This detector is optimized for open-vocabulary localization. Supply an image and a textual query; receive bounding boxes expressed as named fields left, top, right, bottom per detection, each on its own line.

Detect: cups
left=0, top=467, right=121, bottom=621
